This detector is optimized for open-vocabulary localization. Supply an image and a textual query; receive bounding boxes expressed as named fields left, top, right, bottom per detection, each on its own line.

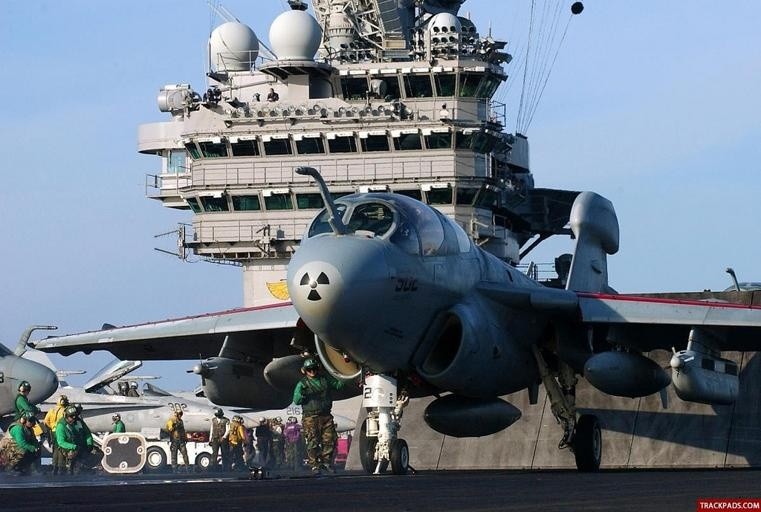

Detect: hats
left=17, top=381, right=32, bottom=393
left=20, top=411, right=41, bottom=424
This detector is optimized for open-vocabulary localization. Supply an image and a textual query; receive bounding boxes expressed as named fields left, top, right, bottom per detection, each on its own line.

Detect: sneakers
left=7, top=467, right=32, bottom=477
left=66, top=466, right=81, bottom=475
left=311, top=465, right=321, bottom=474
left=322, top=463, right=334, bottom=473
left=52, top=464, right=64, bottom=475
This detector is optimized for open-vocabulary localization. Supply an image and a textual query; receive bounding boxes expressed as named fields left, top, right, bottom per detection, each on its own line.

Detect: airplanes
left=27, top=165, right=761, bottom=476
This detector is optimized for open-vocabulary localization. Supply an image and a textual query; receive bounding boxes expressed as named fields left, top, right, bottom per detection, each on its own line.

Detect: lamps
left=223, top=101, right=393, bottom=122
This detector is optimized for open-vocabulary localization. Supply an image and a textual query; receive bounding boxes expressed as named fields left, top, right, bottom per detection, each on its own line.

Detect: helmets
left=259, top=416, right=265, bottom=422
left=271, top=416, right=282, bottom=424
left=303, top=357, right=319, bottom=372
left=287, top=416, right=298, bottom=424
left=215, top=408, right=224, bottom=417
left=174, top=409, right=183, bottom=417
left=231, top=415, right=245, bottom=424
left=63, top=407, right=79, bottom=421
left=111, top=413, right=121, bottom=423
left=131, top=381, right=138, bottom=388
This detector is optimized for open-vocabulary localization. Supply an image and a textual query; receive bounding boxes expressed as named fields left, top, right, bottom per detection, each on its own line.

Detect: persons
left=267, top=88, right=278, bottom=102
left=203, top=89, right=214, bottom=102
left=1, top=359, right=347, bottom=475
